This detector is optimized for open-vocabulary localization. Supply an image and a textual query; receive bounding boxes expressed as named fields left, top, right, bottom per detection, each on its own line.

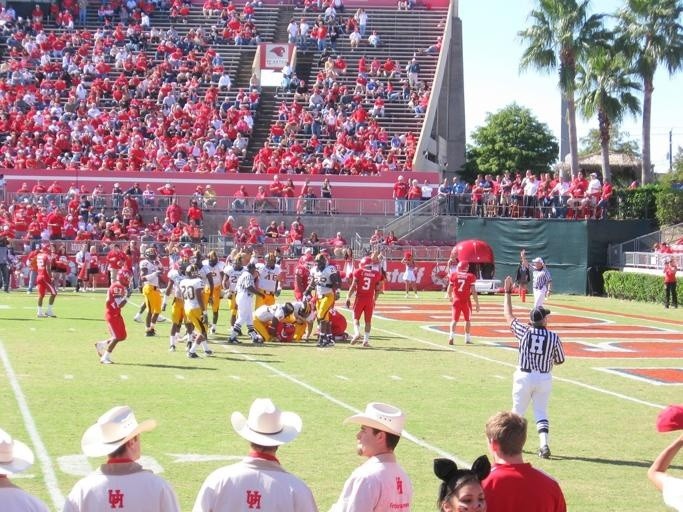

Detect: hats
left=344, top=403, right=407, bottom=439
left=533, top=258, right=543, bottom=263
left=82, top=406, right=156, bottom=457
left=231, top=398, right=302, bottom=446
left=530, top=306, right=550, bottom=321
left=0, top=428, right=35, bottom=475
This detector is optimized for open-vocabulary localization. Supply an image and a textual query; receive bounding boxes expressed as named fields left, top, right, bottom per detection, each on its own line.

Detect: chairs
left=263, top=237, right=349, bottom=244
left=372, top=241, right=456, bottom=260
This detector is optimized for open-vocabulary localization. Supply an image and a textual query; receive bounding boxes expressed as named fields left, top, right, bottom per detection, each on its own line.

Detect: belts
left=520, top=369, right=547, bottom=374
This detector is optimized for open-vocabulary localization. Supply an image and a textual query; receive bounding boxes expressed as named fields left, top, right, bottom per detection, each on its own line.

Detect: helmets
left=118, top=270, right=133, bottom=287
left=145, top=248, right=158, bottom=259
left=457, top=261, right=469, bottom=273
left=178, top=249, right=198, bottom=278
left=359, top=257, right=371, bottom=268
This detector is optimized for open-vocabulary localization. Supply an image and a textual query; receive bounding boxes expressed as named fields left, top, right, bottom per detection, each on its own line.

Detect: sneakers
left=228, top=337, right=241, bottom=344
left=37, top=311, right=57, bottom=318
left=158, top=317, right=165, bottom=321
left=94, top=342, right=102, bottom=356
left=100, top=357, right=112, bottom=364
left=317, top=340, right=334, bottom=348
left=145, top=328, right=157, bottom=336
left=252, top=338, right=261, bottom=342
left=465, top=339, right=472, bottom=344
left=449, top=338, right=454, bottom=344
left=170, top=329, right=215, bottom=358
left=134, top=315, right=143, bottom=323
left=351, top=337, right=359, bottom=345
left=538, top=445, right=550, bottom=457
left=362, top=342, right=369, bottom=346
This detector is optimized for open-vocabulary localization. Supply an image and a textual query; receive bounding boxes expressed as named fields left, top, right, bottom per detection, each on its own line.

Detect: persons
left=1, top=1, right=679, bottom=364
left=504, top=278, right=565, bottom=458
left=0, top=431, right=51, bottom=511
left=645, top=438, right=683, bottom=512
left=64, top=407, right=181, bottom=512
left=323, top=404, right=412, bottom=511
left=192, top=399, right=320, bottom=511
left=482, top=412, right=567, bottom=511
left=433, top=455, right=491, bottom=512
